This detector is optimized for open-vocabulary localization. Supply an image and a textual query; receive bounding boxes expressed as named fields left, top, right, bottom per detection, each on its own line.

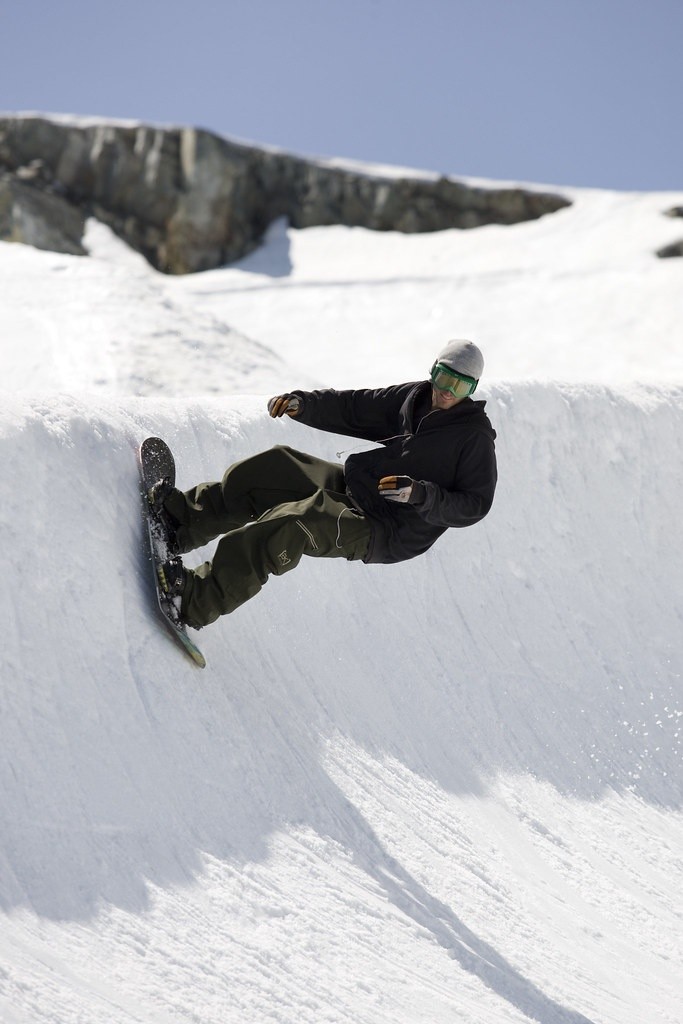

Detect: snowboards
left=137, top=436, right=208, bottom=669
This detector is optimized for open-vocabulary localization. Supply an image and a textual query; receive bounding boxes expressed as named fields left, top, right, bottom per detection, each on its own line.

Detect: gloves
left=378, top=475, right=425, bottom=505
left=267, top=392, right=305, bottom=418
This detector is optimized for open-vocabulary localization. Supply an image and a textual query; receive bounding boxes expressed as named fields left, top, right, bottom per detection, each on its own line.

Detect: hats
left=438, top=339, right=484, bottom=381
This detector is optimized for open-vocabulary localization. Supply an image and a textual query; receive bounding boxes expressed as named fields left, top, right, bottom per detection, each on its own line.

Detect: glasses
left=431, top=364, right=477, bottom=397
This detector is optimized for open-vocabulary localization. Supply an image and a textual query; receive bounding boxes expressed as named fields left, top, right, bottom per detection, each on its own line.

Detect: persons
left=146, top=337, right=499, bottom=631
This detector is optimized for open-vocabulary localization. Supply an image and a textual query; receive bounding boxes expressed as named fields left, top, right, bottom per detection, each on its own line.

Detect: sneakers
left=148, top=479, right=187, bottom=556
left=157, top=557, right=188, bottom=629
